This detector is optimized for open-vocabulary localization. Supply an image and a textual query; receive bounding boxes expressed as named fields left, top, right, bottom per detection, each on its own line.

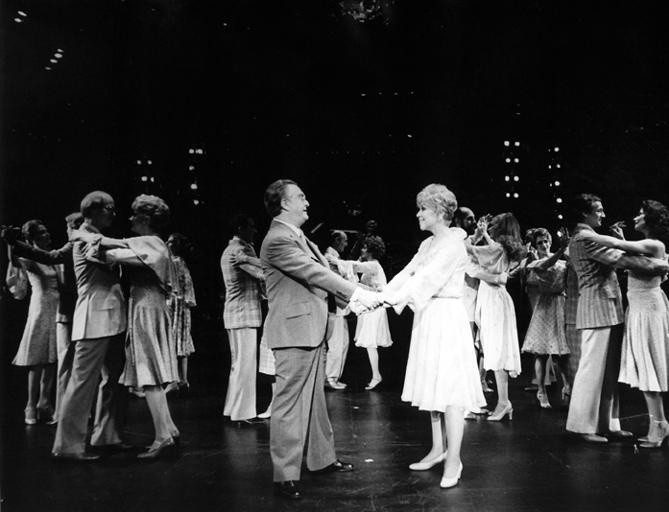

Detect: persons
left=317, top=226, right=393, bottom=392
left=447, top=206, right=581, bottom=420
left=51, top=189, right=126, bottom=464
left=67, top=191, right=180, bottom=462
left=218, top=213, right=284, bottom=427
left=356, top=182, right=489, bottom=471
left=258, top=178, right=388, bottom=501
left=1, top=212, right=196, bottom=433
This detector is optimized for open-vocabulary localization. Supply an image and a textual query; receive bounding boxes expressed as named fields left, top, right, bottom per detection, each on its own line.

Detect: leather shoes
left=324, top=382, right=347, bottom=391
left=464, top=408, right=488, bottom=420
left=24, top=406, right=135, bottom=460
left=605, top=429, right=633, bottom=438
left=277, top=479, right=303, bottom=500
left=315, top=460, right=354, bottom=473
left=525, top=385, right=551, bottom=391
left=566, top=430, right=608, bottom=443
left=223, top=407, right=272, bottom=429
left=636, top=423, right=669, bottom=448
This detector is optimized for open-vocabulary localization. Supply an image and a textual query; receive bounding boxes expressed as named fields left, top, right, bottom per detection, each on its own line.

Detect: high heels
left=145, top=429, right=180, bottom=450
left=365, top=374, right=383, bottom=390
left=536, top=390, right=553, bottom=409
left=137, top=437, right=176, bottom=459
left=409, top=452, right=448, bottom=471
left=486, top=402, right=513, bottom=421
left=440, top=461, right=463, bottom=488
left=561, top=386, right=570, bottom=400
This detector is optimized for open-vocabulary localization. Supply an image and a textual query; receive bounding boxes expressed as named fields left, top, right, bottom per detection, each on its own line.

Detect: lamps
left=330, top=0, right=397, bottom=26
